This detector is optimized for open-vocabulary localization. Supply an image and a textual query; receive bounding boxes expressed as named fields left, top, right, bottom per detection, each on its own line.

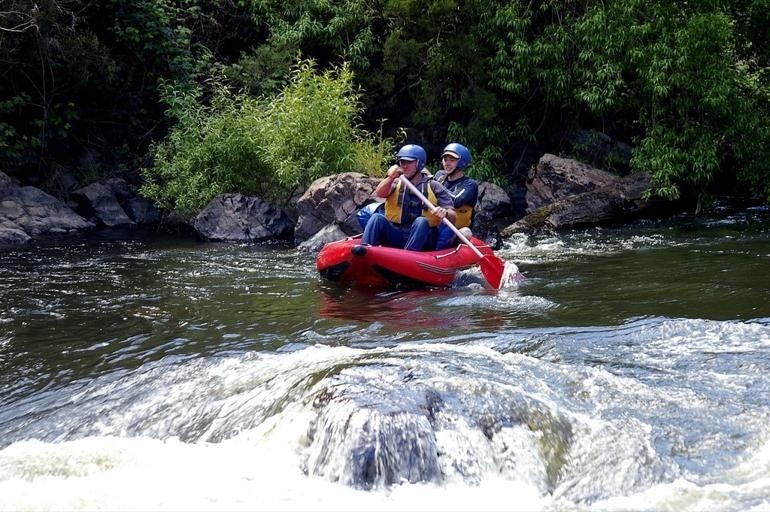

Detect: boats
left=316, top=233, right=494, bottom=289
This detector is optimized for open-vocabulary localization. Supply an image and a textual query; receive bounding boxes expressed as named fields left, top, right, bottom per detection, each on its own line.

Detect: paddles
left=387, top=164, right=504, bottom=288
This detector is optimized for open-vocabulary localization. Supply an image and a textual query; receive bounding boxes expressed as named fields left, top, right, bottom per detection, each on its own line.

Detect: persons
left=361, top=144, right=457, bottom=252
left=430, top=143, right=479, bottom=250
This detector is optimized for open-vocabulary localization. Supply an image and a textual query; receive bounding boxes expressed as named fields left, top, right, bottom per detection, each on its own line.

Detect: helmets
left=396, top=144, right=426, bottom=172
left=440, top=142, right=472, bottom=170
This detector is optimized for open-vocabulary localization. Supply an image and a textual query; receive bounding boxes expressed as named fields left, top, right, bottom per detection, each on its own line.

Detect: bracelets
left=446, top=211, right=449, bottom=217
